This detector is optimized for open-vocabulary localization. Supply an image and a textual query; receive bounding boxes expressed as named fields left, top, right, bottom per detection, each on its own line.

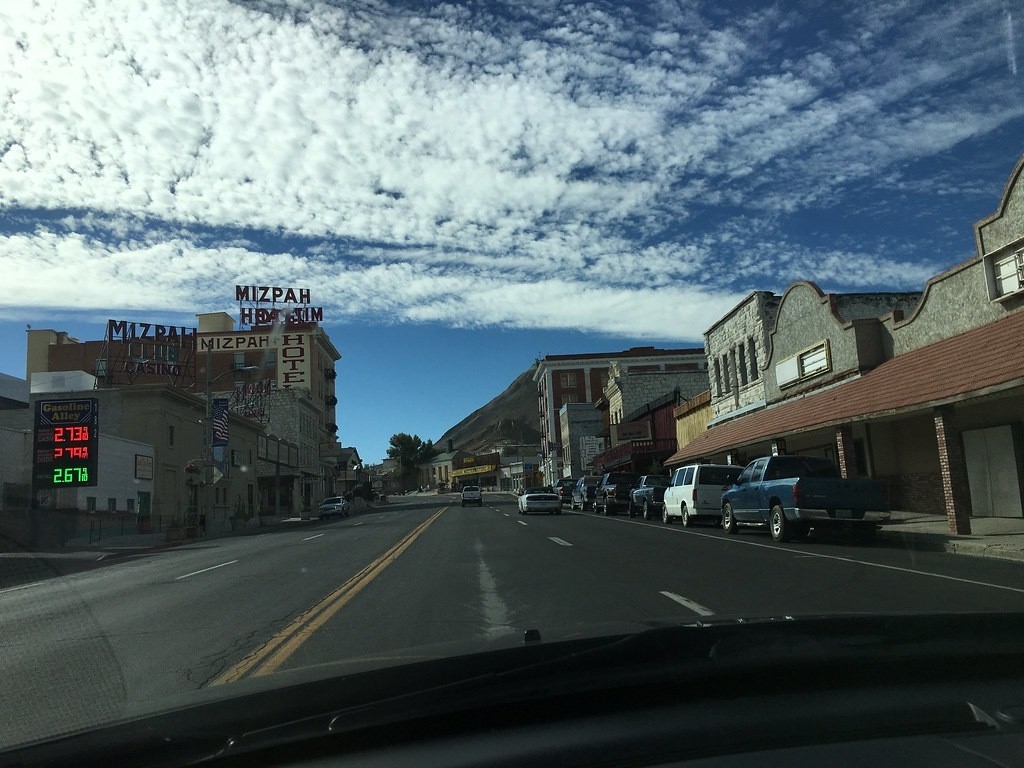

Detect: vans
left=662, top=461, right=746, bottom=526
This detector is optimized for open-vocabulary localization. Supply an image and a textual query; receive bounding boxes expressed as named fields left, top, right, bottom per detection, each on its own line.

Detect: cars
left=517, top=486, right=562, bottom=514
left=319, top=496, right=350, bottom=521
left=0, top=604, right=1024, bottom=767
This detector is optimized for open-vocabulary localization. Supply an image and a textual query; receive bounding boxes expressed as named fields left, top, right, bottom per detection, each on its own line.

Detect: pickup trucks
left=629, top=474, right=673, bottom=520
left=461, top=486, right=482, bottom=507
left=722, top=453, right=876, bottom=543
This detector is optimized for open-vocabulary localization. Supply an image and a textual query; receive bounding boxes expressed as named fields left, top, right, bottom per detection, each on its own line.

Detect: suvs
left=552, top=478, right=579, bottom=504
left=571, top=475, right=603, bottom=511
left=593, top=472, right=639, bottom=516
